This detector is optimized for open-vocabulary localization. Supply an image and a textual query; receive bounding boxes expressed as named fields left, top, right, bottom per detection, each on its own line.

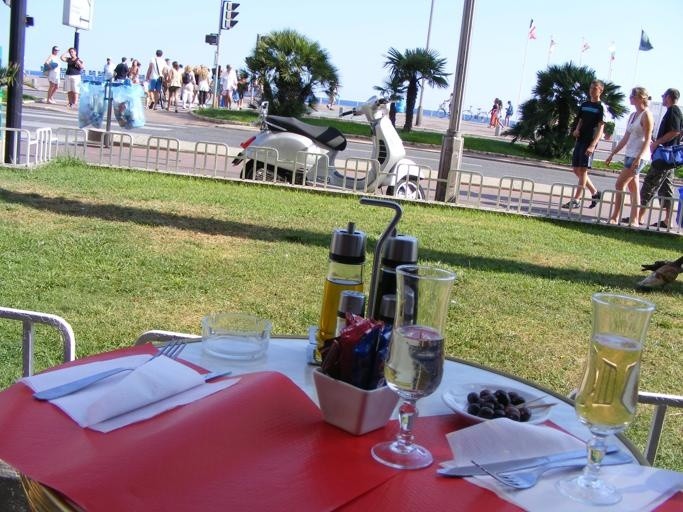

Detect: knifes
left=437, top=442, right=618, bottom=477
left=200, top=369, right=231, bottom=380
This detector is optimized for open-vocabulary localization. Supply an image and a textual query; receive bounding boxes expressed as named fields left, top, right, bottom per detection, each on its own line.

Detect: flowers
left=309, top=305, right=397, bottom=392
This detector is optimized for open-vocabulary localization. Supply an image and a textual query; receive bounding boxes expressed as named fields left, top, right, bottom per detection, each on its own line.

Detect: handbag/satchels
left=160, top=76, right=164, bottom=85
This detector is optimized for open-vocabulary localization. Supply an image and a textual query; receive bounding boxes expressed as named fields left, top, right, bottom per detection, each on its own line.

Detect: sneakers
left=588, top=190, right=602, bottom=209
left=149, top=101, right=155, bottom=109
left=562, top=199, right=581, bottom=209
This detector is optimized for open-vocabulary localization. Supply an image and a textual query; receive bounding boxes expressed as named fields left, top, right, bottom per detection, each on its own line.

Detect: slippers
left=621, top=217, right=645, bottom=225
left=651, top=221, right=669, bottom=228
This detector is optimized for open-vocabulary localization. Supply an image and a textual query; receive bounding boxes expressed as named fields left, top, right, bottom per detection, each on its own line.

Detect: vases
left=308, top=368, right=405, bottom=437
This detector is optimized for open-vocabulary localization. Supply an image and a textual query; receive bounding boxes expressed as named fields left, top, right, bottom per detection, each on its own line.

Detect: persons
left=99, top=49, right=262, bottom=113
left=59, top=47, right=85, bottom=108
left=622, top=88, right=683, bottom=227
left=326, top=78, right=337, bottom=111
left=487, top=98, right=499, bottom=129
left=504, top=100, right=512, bottom=127
left=494, top=100, right=504, bottom=129
left=559, top=79, right=605, bottom=209
left=603, top=87, right=654, bottom=228
left=42, top=46, right=59, bottom=105
left=443, top=94, right=454, bottom=116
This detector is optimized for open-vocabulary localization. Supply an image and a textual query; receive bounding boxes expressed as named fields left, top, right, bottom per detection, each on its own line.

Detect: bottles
left=314, top=226, right=420, bottom=364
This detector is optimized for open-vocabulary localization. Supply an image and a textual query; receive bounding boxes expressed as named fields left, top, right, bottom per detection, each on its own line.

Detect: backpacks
left=652, top=143, right=683, bottom=171
left=182, top=70, right=191, bottom=85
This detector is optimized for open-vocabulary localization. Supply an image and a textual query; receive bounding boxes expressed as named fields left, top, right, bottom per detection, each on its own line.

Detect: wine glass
left=366, top=261, right=458, bottom=478
left=552, top=290, right=657, bottom=509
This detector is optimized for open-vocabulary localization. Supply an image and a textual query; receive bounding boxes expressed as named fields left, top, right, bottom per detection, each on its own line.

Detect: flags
left=581, top=39, right=590, bottom=54
left=528, top=19, right=536, bottom=39
left=550, top=39, right=556, bottom=49
left=638, top=28, right=654, bottom=51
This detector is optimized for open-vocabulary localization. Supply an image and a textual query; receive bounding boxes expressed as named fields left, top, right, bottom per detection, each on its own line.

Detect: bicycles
left=160, top=80, right=167, bottom=110
left=436, top=100, right=487, bottom=123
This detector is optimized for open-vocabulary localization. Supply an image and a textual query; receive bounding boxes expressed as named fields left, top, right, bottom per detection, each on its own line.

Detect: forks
left=32, top=339, right=188, bottom=401
left=469, top=452, right=634, bottom=492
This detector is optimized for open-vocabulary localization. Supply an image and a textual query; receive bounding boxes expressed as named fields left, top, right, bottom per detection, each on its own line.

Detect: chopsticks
left=508, top=394, right=559, bottom=412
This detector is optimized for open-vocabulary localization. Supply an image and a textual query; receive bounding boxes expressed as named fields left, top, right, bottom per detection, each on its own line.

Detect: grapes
left=467, top=389, right=532, bottom=421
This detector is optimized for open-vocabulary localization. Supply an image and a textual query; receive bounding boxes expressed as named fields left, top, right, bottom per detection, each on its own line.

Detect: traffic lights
left=205, top=34, right=215, bottom=44
left=230, top=2, right=239, bottom=29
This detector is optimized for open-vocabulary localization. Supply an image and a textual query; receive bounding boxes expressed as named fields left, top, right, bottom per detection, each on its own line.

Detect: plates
left=439, top=382, right=552, bottom=426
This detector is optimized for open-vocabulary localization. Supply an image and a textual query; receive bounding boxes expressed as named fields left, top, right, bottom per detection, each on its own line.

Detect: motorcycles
left=231, top=84, right=427, bottom=201
